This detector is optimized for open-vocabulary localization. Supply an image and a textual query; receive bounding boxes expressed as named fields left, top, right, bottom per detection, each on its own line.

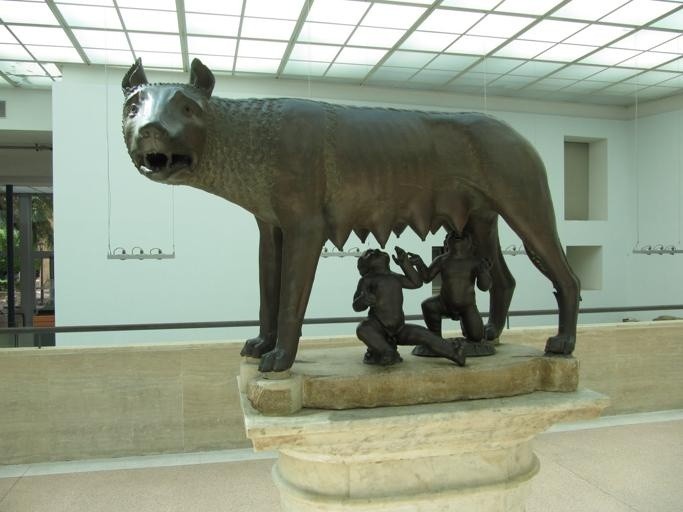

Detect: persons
left=352, top=246, right=467, bottom=367
left=406, top=229, right=492, bottom=342
